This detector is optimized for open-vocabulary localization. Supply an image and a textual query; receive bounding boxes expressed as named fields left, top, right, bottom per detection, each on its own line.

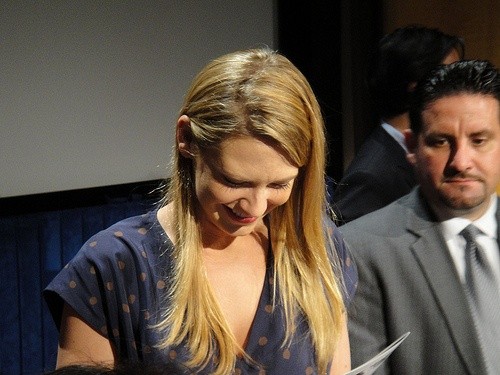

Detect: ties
left=458, top=224, right=500, bottom=375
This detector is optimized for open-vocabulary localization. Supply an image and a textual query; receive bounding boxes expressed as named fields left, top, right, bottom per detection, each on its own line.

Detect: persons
left=329, top=26, right=466, bottom=226
left=334, top=59, right=500, bottom=375
left=44, top=49, right=359, bottom=374
